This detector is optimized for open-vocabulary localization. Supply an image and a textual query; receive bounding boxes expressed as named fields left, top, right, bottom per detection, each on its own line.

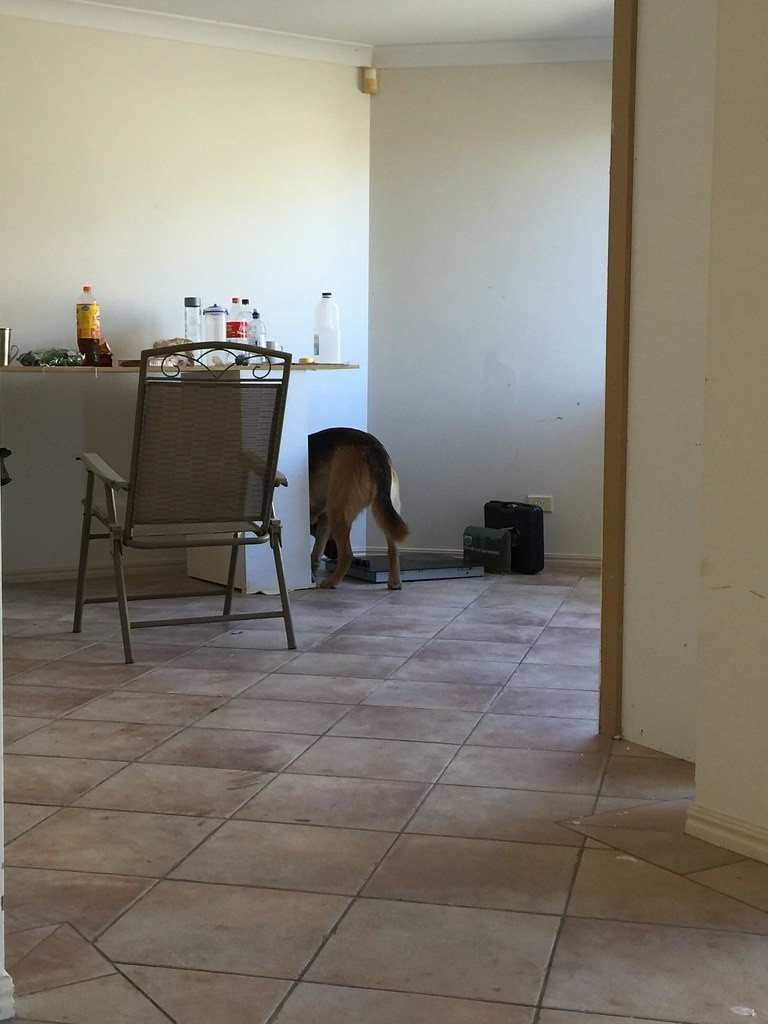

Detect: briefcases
left=484, top=499, right=546, bottom=575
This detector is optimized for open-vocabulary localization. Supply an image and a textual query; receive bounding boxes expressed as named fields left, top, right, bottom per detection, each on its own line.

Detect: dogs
left=307, top=426, right=410, bottom=591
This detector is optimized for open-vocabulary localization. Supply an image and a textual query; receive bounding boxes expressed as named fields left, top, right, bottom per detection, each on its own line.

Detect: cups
left=203, top=304, right=228, bottom=366
left=265, top=340, right=283, bottom=365
left=184, top=296, right=202, bottom=365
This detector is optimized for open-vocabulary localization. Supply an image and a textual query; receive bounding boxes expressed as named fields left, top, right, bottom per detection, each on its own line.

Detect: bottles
left=248, top=307, right=267, bottom=366
left=238, top=298, right=255, bottom=366
left=313, top=293, right=342, bottom=364
left=225, top=297, right=249, bottom=366
left=76, top=286, right=102, bottom=367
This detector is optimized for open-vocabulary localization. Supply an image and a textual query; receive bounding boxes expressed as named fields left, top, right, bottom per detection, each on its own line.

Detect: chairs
left=73, top=340, right=297, bottom=664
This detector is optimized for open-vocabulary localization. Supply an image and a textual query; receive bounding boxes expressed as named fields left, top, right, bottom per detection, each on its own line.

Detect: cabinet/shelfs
left=0, top=363, right=360, bottom=596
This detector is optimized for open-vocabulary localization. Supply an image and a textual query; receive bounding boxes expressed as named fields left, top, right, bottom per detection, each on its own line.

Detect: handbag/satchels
left=463, top=524, right=521, bottom=575
